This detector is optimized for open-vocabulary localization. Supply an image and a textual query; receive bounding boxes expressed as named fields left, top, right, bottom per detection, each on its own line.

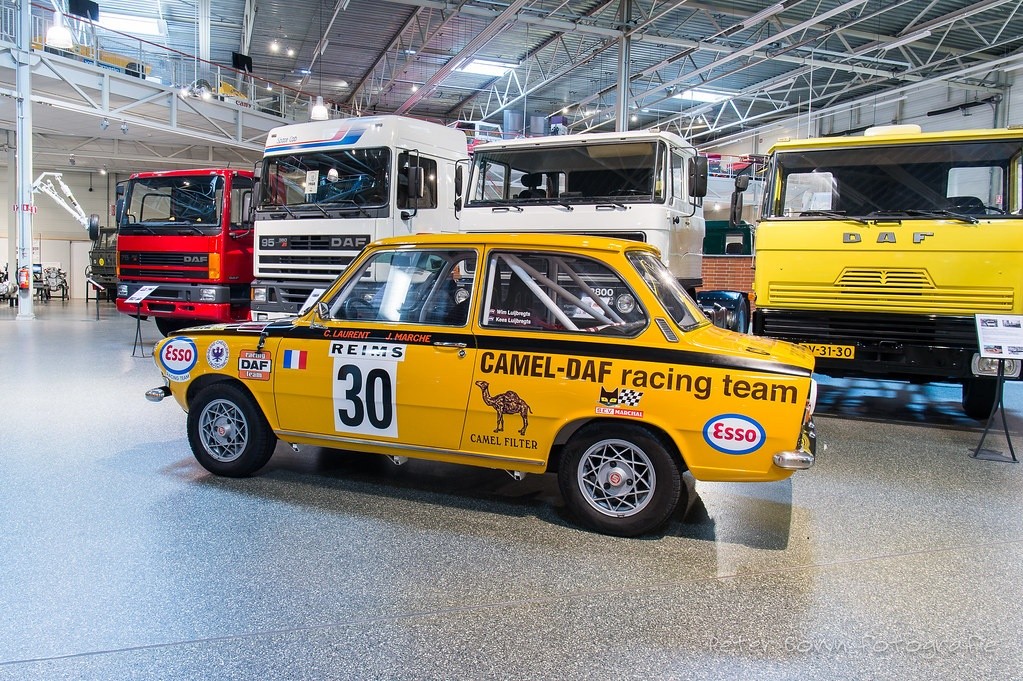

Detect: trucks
left=751, top=125, right=1023, bottom=422
left=84, top=226, right=117, bottom=303
left=113, top=167, right=257, bottom=337
left=248, top=114, right=473, bottom=324
left=451, top=131, right=751, bottom=337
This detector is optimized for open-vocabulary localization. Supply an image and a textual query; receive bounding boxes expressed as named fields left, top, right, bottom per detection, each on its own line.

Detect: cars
left=144, top=228, right=820, bottom=538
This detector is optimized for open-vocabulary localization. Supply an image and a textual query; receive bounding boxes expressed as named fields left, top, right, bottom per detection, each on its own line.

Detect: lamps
left=697, top=116, right=702, bottom=124
left=326, top=153, right=338, bottom=182
left=121, top=122, right=129, bottom=136
left=181, top=85, right=192, bottom=96
left=310, top=0, right=329, bottom=120
left=196, top=87, right=211, bottom=99
left=267, top=83, right=272, bottom=90
left=101, top=166, right=109, bottom=175
left=69, top=156, right=76, bottom=167
left=99, top=119, right=109, bottom=131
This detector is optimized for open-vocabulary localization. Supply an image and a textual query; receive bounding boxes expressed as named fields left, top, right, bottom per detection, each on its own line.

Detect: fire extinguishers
left=15, top=264, right=29, bottom=290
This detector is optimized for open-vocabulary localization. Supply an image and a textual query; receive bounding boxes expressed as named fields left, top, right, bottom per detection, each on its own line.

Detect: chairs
left=517, top=173, right=546, bottom=200
left=932, top=196, right=985, bottom=214
